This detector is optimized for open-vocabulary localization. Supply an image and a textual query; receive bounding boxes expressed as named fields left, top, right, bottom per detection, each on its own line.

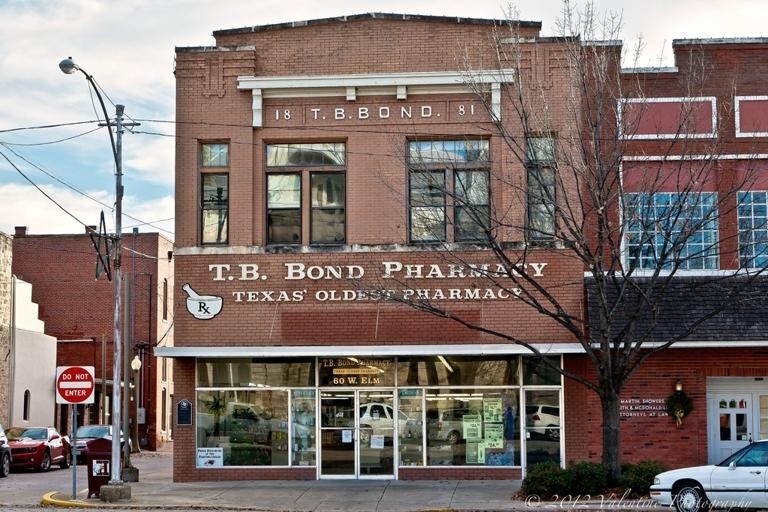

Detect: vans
left=515, top=404, right=559, bottom=441
left=428, top=408, right=486, bottom=445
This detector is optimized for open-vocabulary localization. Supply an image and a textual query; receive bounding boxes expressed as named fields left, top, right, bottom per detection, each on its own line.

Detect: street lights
left=59, top=59, right=123, bottom=484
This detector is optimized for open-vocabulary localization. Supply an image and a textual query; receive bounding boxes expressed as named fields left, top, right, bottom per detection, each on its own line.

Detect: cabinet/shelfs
left=291, top=396, right=316, bottom=466
left=398, top=397, right=423, bottom=466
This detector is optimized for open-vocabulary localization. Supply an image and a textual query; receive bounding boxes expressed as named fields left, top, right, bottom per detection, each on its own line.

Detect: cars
left=334, top=403, right=421, bottom=443
left=0, top=424, right=73, bottom=476
left=649, top=439, right=768, bottom=512
left=71, top=425, right=133, bottom=465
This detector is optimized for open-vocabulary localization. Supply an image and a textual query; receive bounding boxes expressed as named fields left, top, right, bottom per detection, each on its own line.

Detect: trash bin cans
left=80, top=438, right=125, bottom=499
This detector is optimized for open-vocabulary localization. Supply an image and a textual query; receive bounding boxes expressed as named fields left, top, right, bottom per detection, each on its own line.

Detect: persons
left=293, top=402, right=316, bottom=465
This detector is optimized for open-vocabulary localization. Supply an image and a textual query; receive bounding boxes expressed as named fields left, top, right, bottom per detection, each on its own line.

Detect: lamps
left=676, top=379, right=683, bottom=395
left=130, top=352, right=148, bottom=372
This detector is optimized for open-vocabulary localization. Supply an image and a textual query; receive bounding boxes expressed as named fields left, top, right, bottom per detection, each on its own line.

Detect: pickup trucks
left=197, top=402, right=313, bottom=451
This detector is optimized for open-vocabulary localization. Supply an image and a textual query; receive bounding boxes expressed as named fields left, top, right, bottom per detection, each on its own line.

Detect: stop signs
left=55, top=365, right=96, bottom=405
